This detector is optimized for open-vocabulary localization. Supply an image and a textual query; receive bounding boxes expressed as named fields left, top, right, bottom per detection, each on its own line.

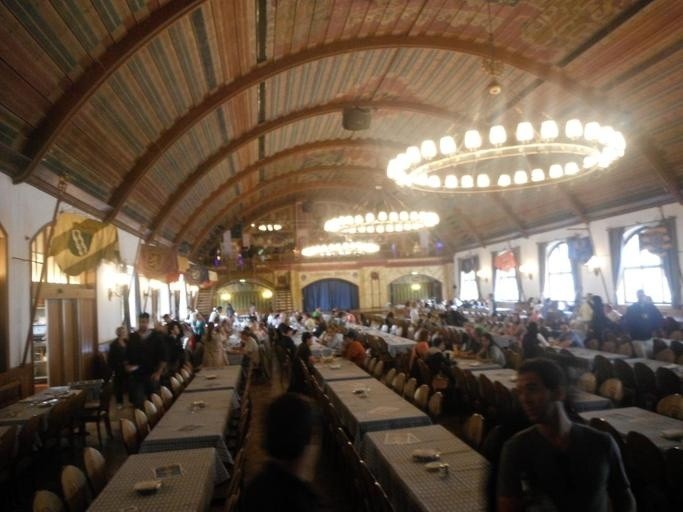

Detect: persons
left=488, top=352, right=640, bottom=510
left=229, top=389, right=327, bottom=512
left=105, top=285, right=682, bottom=413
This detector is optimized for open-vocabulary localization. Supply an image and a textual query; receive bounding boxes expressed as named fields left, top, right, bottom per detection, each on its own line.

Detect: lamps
left=298, top=231, right=382, bottom=261
left=383, top=0, right=629, bottom=197
left=318, top=182, right=442, bottom=238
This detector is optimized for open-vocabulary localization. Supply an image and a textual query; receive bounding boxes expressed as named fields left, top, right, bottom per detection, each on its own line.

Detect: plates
left=351, top=388, right=367, bottom=398
left=132, top=480, right=161, bottom=497
left=152, top=464, right=179, bottom=479
left=661, top=427, right=683, bottom=451
left=205, top=374, right=214, bottom=380
left=190, top=399, right=208, bottom=413
left=328, top=364, right=341, bottom=369
left=509, top=375, right=518, bottom=381
left=412, top=449, right=448, bottom=478
